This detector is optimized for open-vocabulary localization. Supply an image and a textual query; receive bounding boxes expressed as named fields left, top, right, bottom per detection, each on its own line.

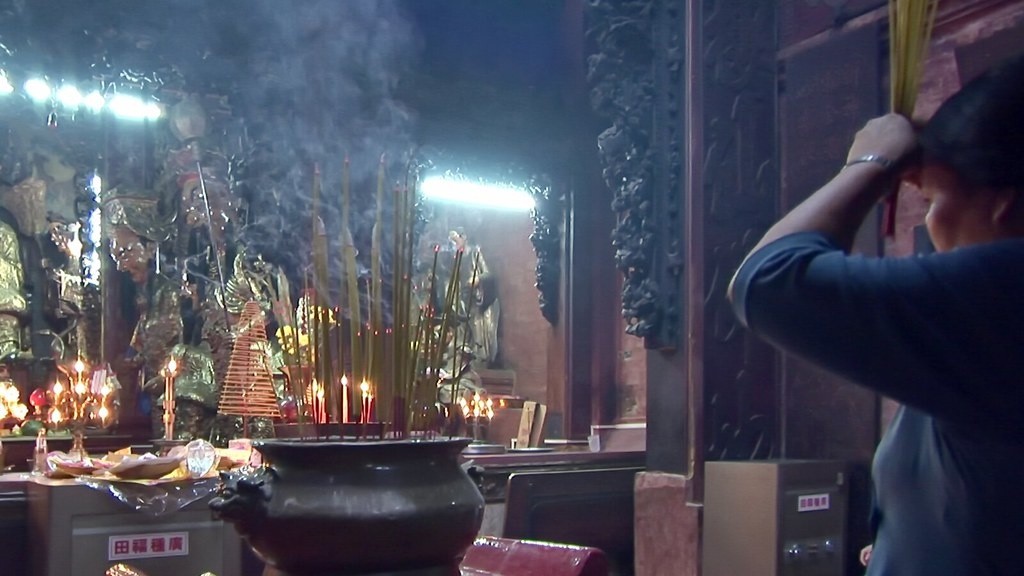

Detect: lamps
left=50, top=353, right=119, bottom=457
left=458, top=390, right=506, bottom=440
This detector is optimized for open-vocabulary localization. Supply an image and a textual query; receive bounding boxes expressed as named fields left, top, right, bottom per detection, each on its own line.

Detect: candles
left=163, top=360, right=177, bottom=439
left=310, top=374, right=377, bottom=424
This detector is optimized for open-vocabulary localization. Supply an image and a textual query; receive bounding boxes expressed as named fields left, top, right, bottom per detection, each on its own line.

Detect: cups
left=587, top=434, right=600, bottom=452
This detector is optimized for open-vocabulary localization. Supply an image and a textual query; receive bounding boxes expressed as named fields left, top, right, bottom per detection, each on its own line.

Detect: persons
left=0, top=152, right=291, bottom=443
left=727, top=53, right=1024, bottom=576
left=447, top=226, right=500, bottom=362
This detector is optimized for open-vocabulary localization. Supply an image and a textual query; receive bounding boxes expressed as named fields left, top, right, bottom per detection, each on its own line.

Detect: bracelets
left=840, top=156, right=893, bottom=170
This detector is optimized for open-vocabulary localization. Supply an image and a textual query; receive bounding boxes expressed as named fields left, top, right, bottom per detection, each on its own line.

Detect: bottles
left=32, top=427, right=48, bottom=476
